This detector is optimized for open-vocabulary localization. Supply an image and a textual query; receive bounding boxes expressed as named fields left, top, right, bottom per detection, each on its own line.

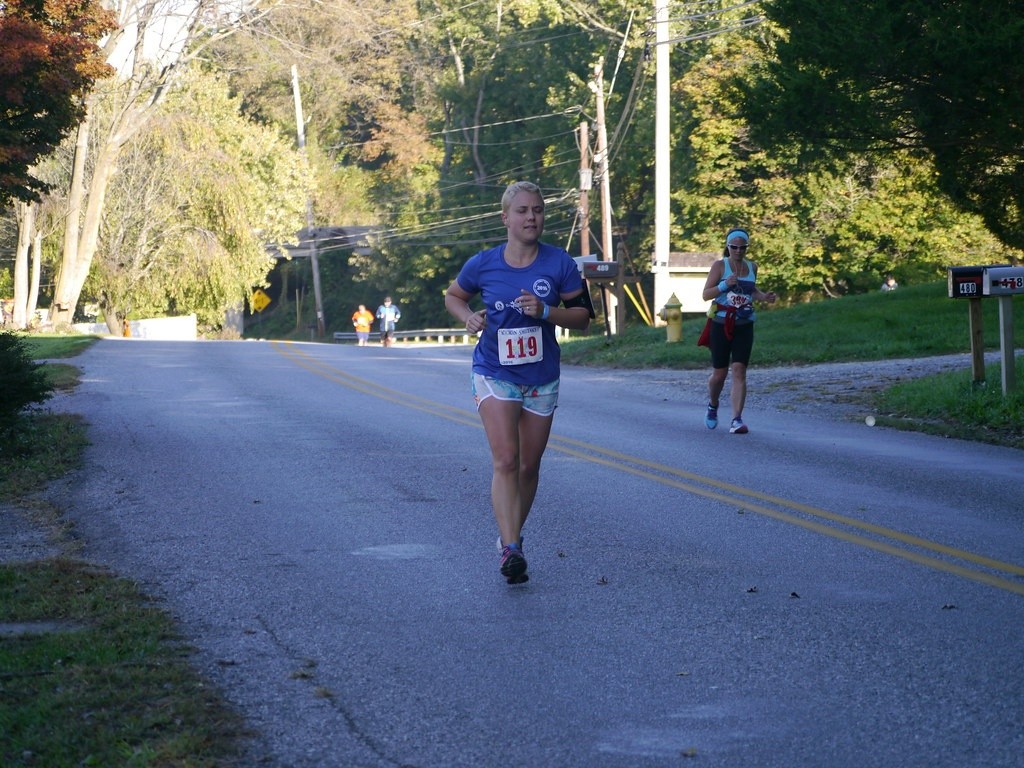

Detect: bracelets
left=540, top=304, right=549, bottom=319
left=718, top=280, right=729, bottom=292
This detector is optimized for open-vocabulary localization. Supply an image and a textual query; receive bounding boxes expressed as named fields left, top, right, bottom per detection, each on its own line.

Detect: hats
left=384, top=297, right=392, bottom=302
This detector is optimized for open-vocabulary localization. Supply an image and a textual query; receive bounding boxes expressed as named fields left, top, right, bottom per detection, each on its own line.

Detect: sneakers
left=497, top=536, right=529, bottom=584
left=729, top=416, right=748, bottom=434
left=705, top=400, right=720, bottom=429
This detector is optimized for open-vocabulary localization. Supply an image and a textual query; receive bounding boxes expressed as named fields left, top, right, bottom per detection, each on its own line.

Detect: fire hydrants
left=655, top=291, right=683, bottom=343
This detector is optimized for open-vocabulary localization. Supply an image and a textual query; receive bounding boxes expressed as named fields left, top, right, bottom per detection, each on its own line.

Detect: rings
left=527, top=307, right=530, bottom=310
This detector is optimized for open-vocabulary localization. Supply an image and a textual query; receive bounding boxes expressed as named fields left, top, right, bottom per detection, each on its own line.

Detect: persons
left=881, top=275, right=898, bottom=292
left=352, top=304, right=374, bottom=346
left=118, top=318, right=130, bottom=337
left=445, top=182, right=590, bottom=584
left=697, top=229, right=776, bottom=434
left=376, top=296, right=401, bottom=347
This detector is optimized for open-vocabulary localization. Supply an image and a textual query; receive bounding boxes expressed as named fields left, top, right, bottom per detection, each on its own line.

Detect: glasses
left=728, top=244, right=748, bottom=250
